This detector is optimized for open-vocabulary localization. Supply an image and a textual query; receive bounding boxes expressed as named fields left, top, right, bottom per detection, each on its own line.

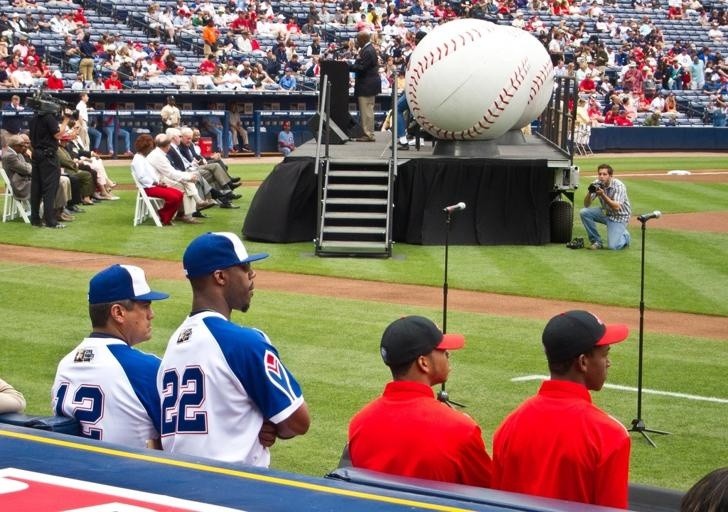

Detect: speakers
left=307, top=111, right=349, bottom=144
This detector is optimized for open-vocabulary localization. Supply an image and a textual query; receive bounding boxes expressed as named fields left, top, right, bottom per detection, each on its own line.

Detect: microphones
left=442, top=201, right=466, bottom=215
left=330, top=45, right=349, bottom=54
left=637, top=211, right=661, bottom=222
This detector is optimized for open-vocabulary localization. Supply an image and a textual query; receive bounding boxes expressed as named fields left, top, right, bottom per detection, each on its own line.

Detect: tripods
left=627, top=221, right=673, bottom=448
left=437, top=214, right=465, bottom=408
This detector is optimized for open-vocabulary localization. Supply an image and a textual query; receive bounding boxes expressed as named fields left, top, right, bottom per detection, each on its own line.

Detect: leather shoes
left=41, top=181, right=120, bottom=229
left=182, top=178, right=244, bottom=224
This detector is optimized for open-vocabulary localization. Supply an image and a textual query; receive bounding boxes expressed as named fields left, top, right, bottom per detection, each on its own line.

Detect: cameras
left=588, top=182, right=605, bottom=193
left=567, top=238, right=584, bottom=249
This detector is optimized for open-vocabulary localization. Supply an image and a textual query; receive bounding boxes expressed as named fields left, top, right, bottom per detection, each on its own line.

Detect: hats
left=541, top=306, right=632, bottom=357
left=377, top=312, right=466, bottom=366
left=181, top=229, right=271, bottom=279
left=87, top=262, right=170, bottom=308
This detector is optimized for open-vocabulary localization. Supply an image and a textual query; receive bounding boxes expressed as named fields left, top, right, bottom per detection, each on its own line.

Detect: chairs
left=0, top=163, right=177, bottom=226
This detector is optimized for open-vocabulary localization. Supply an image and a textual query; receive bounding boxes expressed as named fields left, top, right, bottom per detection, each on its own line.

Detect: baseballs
left=404, top=18, right=554, bottom=140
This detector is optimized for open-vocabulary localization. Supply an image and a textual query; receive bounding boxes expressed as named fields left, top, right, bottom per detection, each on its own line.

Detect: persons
left=279, top=121, right=295, bottom=155
left=49, top=263, right=169, bottom=451
left=1, top=93, right=133, bottom=228
left=0, top=378, right=26, bottom=414
left=155, top=229, right=311, bottom=471
left=2, top=0, right=728, bottom=126
left=346, top=315, right=494, bottom=489
left=580, top=164, right=630, bottom=249
left=132, top=95, right=251, bottom=223
left=490, top=308, right=631, bottom=509
left=347, top=31, right=381, bottom=142
left=680, top=467, right=728, bottom=512
